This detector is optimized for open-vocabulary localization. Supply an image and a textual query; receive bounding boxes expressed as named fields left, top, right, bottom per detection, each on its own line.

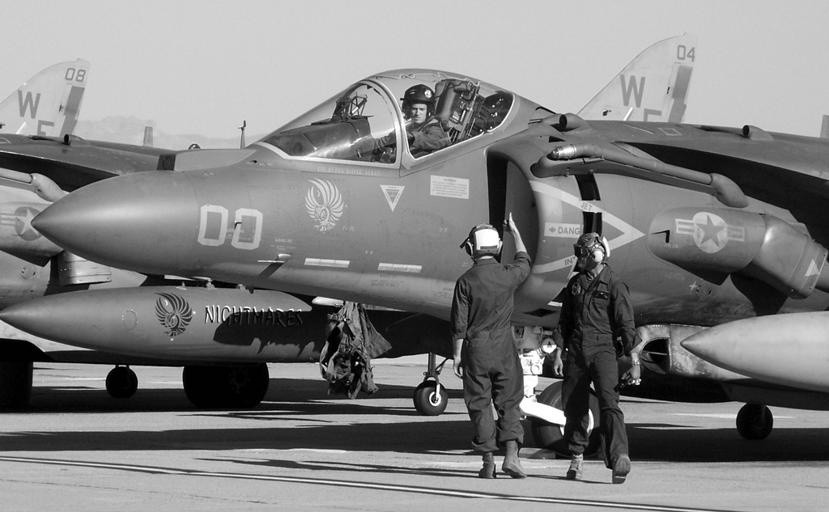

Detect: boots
left=612, top=455, right=631, bottom=484
left=566, top=453, right=583, bottom=480
left=502, top=440, right=527, bottom=478
left=479, top=452, right=496, bottom=478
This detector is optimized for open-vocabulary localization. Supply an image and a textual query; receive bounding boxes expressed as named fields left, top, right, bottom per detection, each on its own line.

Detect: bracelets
left=631, top=359, right=641, bottom=367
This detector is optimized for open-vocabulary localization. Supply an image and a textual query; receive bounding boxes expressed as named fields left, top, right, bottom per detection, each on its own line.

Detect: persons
left=449, top=209, right=534, bottom=481
left=550, top=231, right=645, bottom=484
left=374, top=84, right=453, bottom=162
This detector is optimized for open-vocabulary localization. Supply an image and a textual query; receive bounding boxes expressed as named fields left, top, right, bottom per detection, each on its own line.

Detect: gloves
left=617, top=361, right=642, bottom=392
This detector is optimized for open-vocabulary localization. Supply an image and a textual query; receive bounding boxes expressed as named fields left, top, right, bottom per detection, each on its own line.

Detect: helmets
left=465, top=223, right=503, bottom=256
left=574, top=232, right=610, bottom=262
left=401, top=84, right=436, bottom=112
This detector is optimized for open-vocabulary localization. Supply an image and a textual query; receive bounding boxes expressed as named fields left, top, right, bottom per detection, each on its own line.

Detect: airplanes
left=0, top=32, right=829, bottom=459
